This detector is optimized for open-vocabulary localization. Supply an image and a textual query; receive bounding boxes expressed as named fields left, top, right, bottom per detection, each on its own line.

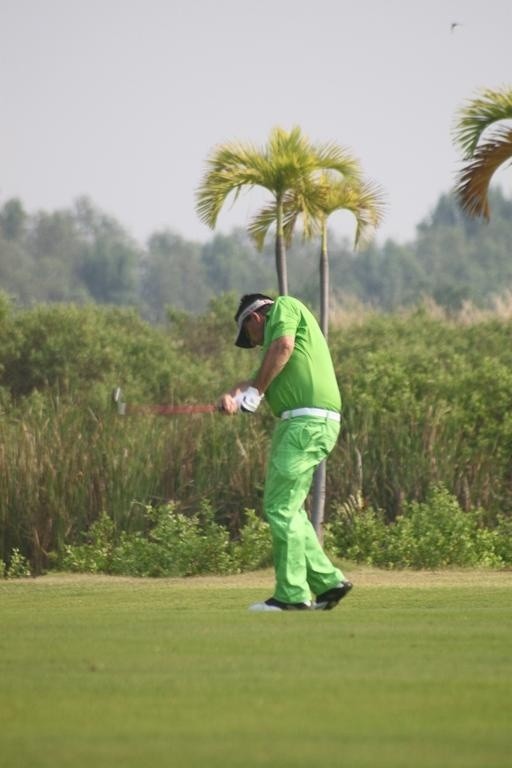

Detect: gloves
left=235, top=386, right=264, bottom=413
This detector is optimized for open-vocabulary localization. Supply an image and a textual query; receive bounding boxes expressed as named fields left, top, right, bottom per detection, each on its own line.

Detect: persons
left=215, top=293, right=352, bottom=611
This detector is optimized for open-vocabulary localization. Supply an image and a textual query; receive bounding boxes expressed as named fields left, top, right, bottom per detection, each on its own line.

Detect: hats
left=235, top=295, right=275, bottom=348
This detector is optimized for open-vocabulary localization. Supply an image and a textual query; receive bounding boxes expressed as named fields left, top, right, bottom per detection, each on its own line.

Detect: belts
left=280, top=407, right=341, bottom=421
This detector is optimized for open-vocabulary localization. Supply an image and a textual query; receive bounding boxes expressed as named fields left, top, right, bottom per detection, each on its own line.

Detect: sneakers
left=315, top=579, right=353, bottom=610
left=252, top=597, right=315, bottom=613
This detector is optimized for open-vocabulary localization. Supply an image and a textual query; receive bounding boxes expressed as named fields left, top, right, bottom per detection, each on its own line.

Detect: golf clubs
left=111, top=386, right=221, bottom=411
